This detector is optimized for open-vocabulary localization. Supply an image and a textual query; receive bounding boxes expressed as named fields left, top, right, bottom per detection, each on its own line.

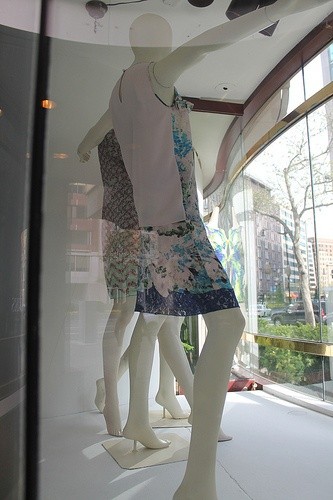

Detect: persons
left=81, top=127, right=191, bottom=437
left=74, top=0, right=329, bottom=500
left=120, top=224, right=233, bottom=452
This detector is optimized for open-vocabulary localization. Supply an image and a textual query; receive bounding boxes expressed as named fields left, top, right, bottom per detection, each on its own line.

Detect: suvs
left=270, top=300, right=332, bottom=327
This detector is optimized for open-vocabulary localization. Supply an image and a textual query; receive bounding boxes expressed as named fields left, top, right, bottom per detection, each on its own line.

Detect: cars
left=246, top=302, right=272, bottom=318
